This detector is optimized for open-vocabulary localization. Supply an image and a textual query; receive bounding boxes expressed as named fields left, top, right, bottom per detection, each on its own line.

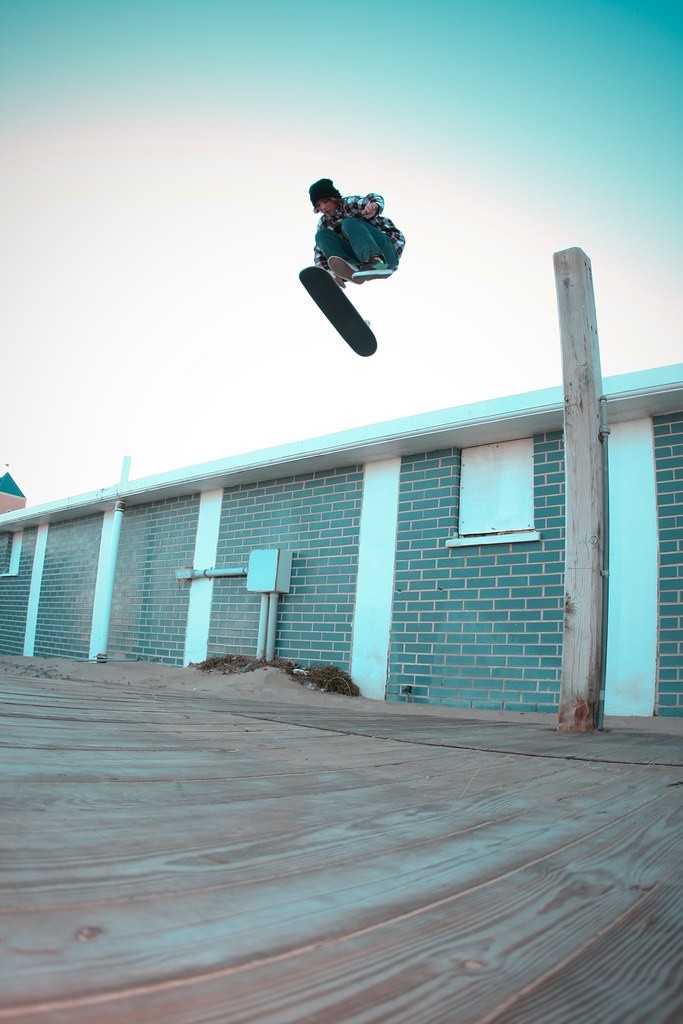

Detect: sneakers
left=352, top=259, right=392, bottom=279
left=328, top=256, right=365, bottom=284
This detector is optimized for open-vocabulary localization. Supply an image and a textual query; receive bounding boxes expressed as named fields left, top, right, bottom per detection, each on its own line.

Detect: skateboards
left=299, top=266, right=378, bottom=357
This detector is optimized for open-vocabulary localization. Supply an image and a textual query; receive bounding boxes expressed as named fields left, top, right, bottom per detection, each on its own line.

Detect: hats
left=309, top=179, right=341, bottom=206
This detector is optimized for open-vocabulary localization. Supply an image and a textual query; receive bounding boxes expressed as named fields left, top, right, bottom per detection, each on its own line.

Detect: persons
left=308, top=177, right=405, bottom=288
